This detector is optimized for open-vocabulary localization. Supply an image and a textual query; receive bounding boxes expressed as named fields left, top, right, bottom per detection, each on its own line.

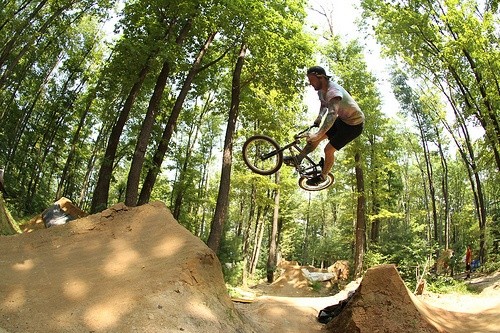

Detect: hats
left=307, top=66, right=332, bottom=78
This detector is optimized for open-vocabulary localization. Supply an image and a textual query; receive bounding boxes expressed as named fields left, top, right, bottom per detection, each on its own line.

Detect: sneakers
left=306, top=173, right=328, bottom=186
left=283, top=155, right=302, bottom=166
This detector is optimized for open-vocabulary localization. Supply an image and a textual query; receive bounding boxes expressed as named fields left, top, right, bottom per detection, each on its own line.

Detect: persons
left=284, top=66, right=366, bottom=186
left=464, top=245, right=472, bottom=280
left=472, top=259, right=480, bottom=272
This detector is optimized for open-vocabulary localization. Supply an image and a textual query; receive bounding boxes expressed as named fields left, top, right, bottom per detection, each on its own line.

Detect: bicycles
left=242, top=123, right=334, bottom=191
